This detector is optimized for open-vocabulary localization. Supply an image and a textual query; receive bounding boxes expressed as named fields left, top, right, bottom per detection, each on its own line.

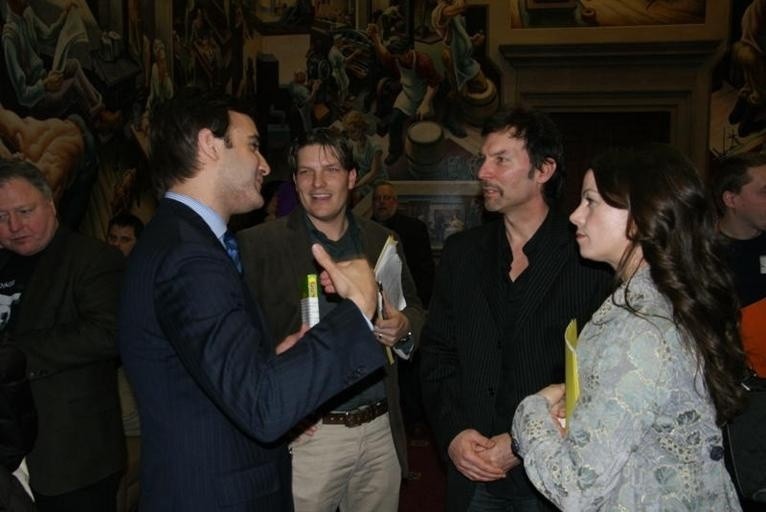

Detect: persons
left=119, top=95, right=386, bottom=511
left=0, top=0, right=485, bottom=193
left=411, top=106, right=623, bottom=510
left=712, top=153, right=765, bottom=510
left=368, top=182, right=435, bottom=436
left=512, top=142, right=760, bottom=512
left=233, top=128, right=423, bottom=511
left=1, top=158, right=145, bottom=510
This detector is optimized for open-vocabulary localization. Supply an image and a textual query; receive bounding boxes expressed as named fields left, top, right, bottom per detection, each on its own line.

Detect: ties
left=224, top=230, right=243, bottom=277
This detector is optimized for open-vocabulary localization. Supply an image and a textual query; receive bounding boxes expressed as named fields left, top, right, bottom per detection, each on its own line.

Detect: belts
left=321, top=400, right=388, bottom=426
